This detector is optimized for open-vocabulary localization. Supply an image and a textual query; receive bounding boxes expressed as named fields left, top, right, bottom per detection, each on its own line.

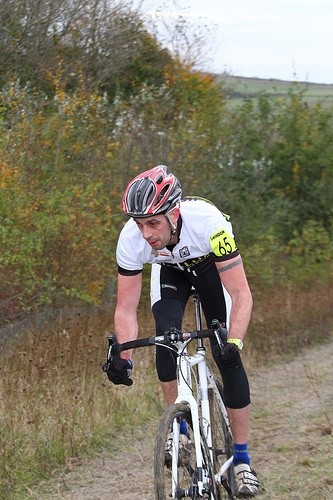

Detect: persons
left=102, top=164, right=261, bottom=497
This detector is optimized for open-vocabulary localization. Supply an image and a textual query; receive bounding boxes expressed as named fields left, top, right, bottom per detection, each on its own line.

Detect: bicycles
left=102, top=282, right=263, bottom=499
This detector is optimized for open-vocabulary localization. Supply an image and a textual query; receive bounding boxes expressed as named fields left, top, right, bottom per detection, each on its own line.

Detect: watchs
left=226, top=337, right=245, bottom=351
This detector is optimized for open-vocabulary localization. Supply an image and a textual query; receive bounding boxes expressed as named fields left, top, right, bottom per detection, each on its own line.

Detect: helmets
left=121, top=165, right=183, bottom=218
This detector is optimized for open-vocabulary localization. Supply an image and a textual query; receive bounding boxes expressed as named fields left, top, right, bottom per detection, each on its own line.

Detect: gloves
left=102, top=357, right=133, bottom=386
left=213, top=343, right=242, bottom=371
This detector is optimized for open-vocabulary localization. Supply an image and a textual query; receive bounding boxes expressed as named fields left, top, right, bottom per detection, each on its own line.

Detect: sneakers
left=233, top=463, right=261, bottom=498
left=164, top=432, right=192, bottom=466
